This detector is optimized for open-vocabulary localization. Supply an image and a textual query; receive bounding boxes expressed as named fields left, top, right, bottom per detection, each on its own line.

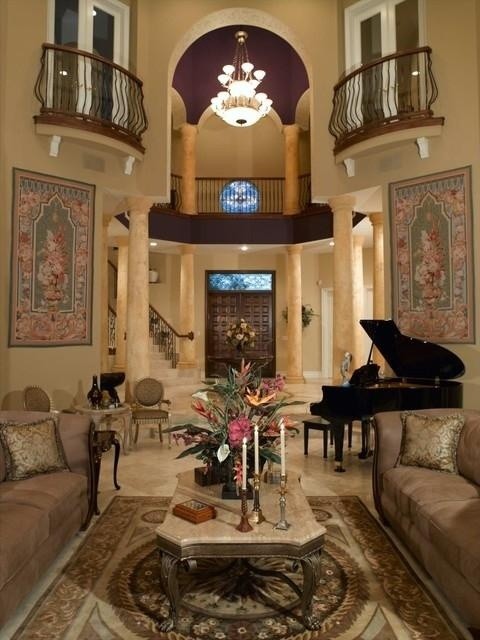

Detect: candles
left=254, top=425, right=260, bottom=474
left=242, top=436, right=247, bottom=489
left=280, top=418, right=287, bottom=476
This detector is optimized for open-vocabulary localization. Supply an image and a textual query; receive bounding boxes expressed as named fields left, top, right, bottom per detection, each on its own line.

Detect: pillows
left=396, top=411, right=466, bottom=475
left=0, top=414, right=68, bottom=480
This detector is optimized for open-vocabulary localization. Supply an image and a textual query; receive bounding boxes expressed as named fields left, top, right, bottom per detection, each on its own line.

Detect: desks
left=154, top=467, right=329, bottom=634
left=209, top=351, right=275, bottom=384
left=66, top=403, right=131, bottom=515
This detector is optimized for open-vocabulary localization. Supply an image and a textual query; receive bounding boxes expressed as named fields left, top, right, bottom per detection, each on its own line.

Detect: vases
left=201, top=452, right=270, bottom=501
left=147, top=269, right=158, bottom=284
left=233, top=344, right=247, bottom=357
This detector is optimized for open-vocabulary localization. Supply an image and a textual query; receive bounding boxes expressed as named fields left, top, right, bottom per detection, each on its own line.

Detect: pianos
left=309, top=318, right=465, bottom=472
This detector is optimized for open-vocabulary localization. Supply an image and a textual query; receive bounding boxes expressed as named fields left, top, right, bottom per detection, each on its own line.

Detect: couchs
left=4, top=412, right=97, bottom=640
left=368, top=406, right=480, bottom=640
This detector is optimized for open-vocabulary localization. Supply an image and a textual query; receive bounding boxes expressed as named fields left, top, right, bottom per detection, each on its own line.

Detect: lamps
left=210, top=30, right=273, bottom=133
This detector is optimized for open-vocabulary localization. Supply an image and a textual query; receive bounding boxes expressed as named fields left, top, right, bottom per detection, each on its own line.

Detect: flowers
left=167, top=354, right=303, bottom=471
left=222, top=317, right=258, bottom=351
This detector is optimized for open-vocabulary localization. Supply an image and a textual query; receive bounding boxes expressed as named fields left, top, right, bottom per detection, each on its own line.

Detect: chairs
left=128, top=377, right=173, bottom=448
left=21, top=384, right=60, bottom=417
left=4, top=389, right=31, bottom=419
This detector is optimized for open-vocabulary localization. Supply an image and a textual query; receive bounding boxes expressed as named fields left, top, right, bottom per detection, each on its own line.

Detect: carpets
left=8, top=494, right=469, bottom=637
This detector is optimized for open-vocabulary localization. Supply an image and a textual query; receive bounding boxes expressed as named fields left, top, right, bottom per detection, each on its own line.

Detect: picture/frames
left=386, top=165, right=478, bottom=347
left=7, top=166, right=96, bottom=348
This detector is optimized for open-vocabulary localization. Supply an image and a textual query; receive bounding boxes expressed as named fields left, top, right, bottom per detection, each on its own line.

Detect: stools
left=301, top=414, right=332, bottom=459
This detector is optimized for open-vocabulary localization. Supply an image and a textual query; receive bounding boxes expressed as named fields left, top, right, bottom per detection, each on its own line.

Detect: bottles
left=87, top=376, right=103, bottom=410
